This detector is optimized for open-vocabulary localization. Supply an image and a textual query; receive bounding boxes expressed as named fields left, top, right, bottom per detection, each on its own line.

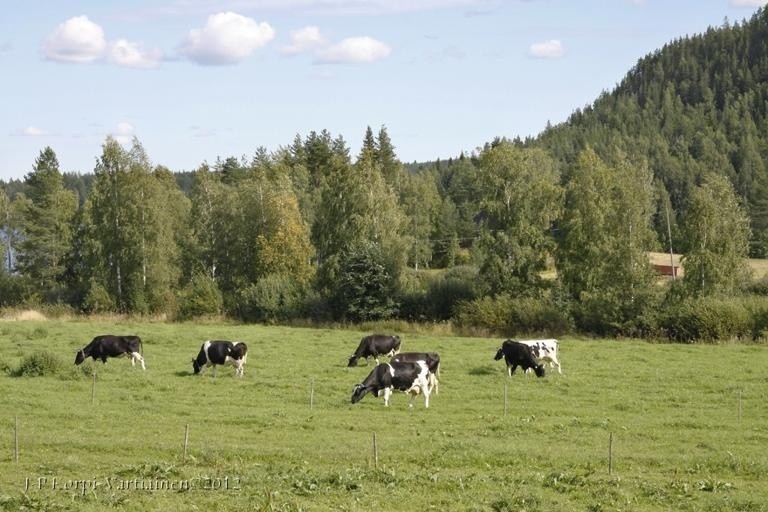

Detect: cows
left=390, top=351, right=440, bottom=396
left=502, top=340, right=546, bottom=378
left=347, top=334, right=401, bottom=367
left=192, top=340, right=248, bottom=378
left=493, top=338, right=562, bottom=375
left=350, top=359, right=431, bottom=408
left=74, top=335, right=146, bottom=370
